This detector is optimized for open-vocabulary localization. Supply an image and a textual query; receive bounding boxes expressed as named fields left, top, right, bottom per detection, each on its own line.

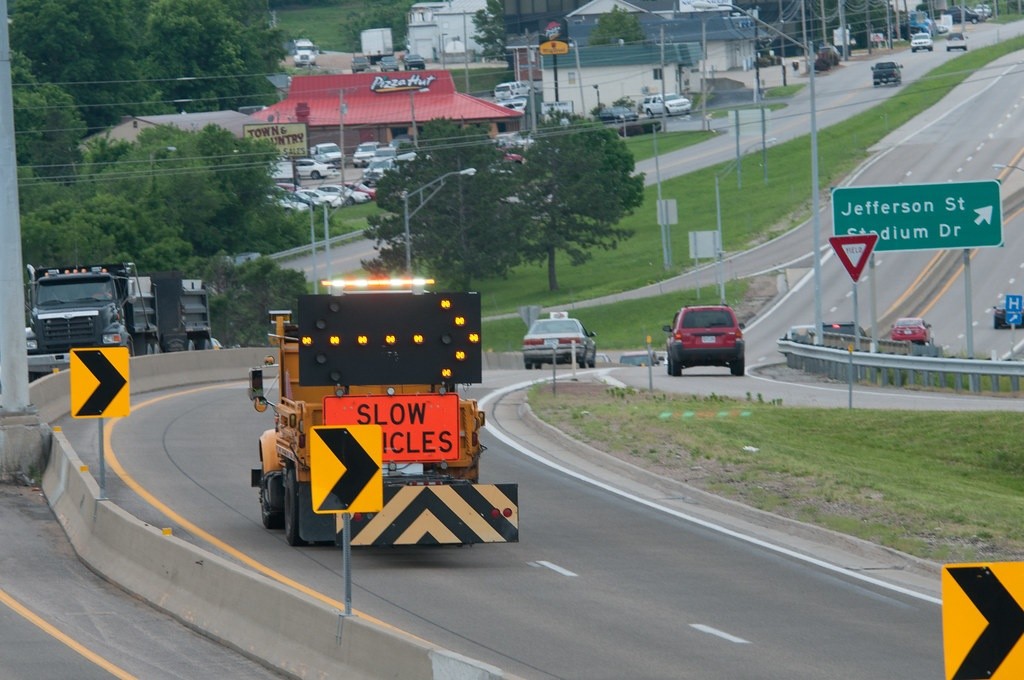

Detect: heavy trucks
left=23, top=263, right=213, bottom=378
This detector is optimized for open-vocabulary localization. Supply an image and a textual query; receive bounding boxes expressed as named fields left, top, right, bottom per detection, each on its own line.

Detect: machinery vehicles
left=247, top=276, right=519, bottom=548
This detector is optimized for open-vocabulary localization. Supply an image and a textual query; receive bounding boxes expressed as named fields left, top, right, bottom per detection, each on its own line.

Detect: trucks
left=361, top=27, right=397, bottom=64
left=294, top=39, right=319, bottom=65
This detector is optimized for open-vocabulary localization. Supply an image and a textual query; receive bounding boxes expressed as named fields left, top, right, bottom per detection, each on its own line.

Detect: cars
left=891, top=318, right=934, bottom=345
left=522, top=311, right=597, bottom=369
left=404, top=54, right=425, bottom=70
left=992, top=299, right=1024, bottom=329
left=942, top=4, right=993, bottom=24
left=350, top=56, right=370, bottom=73
left=379, top=56, right=398, bottom=71
left=274, top=80, right=689, bottom=217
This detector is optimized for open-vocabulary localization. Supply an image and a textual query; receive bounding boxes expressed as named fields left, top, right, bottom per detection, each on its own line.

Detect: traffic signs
left=831, top=180, right=1004, bottom=252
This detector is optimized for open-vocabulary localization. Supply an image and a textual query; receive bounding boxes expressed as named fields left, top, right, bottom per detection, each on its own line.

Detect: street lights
left=399, top=166, right=478, bottom=273
left=690, top=1, right=822, bottom=345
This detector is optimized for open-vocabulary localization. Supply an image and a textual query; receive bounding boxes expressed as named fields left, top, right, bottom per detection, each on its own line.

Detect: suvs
left=910, top=33, right=933, bottom=52
left=822, top=322, right=869, bottom=338
left=946, top=32, right=968, bottom=52
left=870, top=62, right=904, bottom=88
left=662, top=302, right=745, bottom=377
